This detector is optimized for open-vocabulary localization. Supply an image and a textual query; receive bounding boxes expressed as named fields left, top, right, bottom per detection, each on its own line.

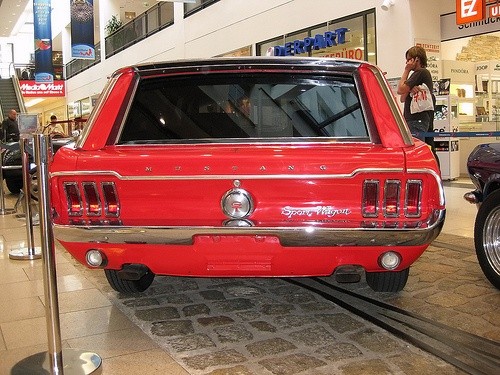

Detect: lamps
left=381, top=0, right=395, bottom=11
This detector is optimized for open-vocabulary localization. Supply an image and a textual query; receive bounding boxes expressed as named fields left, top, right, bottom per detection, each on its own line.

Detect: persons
left=20, top=68, right=29, bottom=80
left=394, top=44, right=434, bottom=141
left=44, top=115, right=66, bottom=137
left=2, top=108, right=21, bottom=146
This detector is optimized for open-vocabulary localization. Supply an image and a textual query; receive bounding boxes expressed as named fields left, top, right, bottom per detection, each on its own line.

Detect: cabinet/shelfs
left=432, top=96, right=460, bottom=181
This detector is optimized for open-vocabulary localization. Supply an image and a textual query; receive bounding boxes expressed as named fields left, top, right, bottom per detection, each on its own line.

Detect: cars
left=1, top=137, right=76, bottom=194
left=463, top=143, right=500, bottom=291
left=50, top=58, right=447, bottom=294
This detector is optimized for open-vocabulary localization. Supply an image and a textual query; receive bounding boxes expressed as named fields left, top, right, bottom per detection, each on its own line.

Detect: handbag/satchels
left=410, top=83, right=434, bottom=115
